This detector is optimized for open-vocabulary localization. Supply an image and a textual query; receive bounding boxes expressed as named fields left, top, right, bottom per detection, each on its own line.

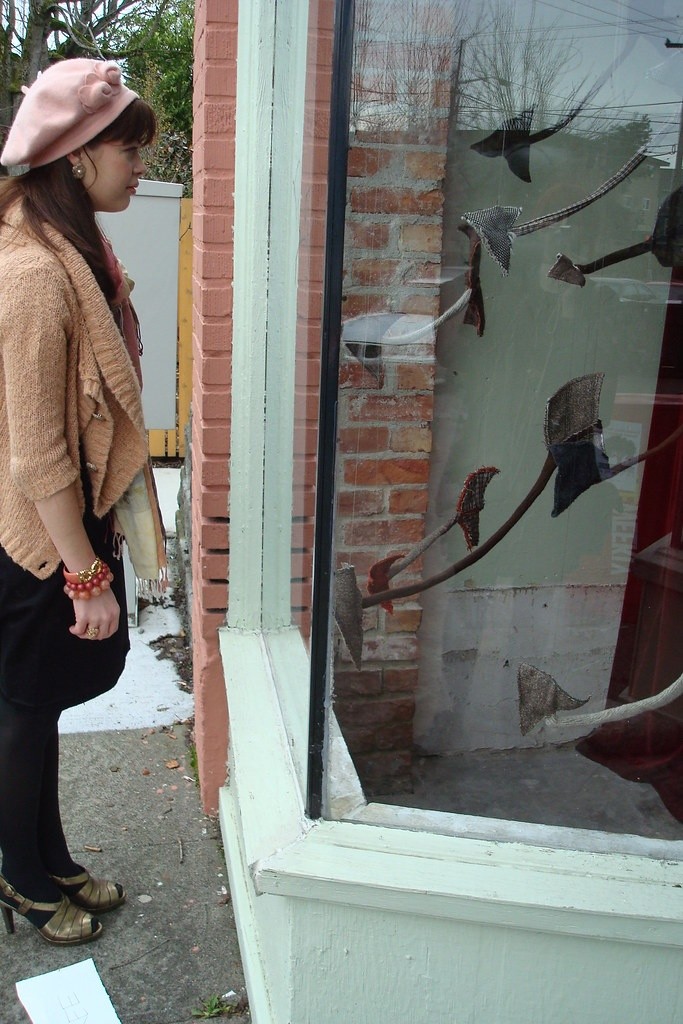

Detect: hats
left=0, top=57, right=140, bottom=179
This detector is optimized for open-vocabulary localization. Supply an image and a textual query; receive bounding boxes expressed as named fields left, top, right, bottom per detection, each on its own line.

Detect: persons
left=0, top=59, right=160, bottom=944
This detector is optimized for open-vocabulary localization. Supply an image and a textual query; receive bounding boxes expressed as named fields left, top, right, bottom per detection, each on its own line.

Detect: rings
left=86, top=628, right=99, bottom=637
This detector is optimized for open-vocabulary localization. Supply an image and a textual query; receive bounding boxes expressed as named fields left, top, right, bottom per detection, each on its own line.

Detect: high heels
left=0, top=871, right=103, bottom=946
left=46, top=861, right=127, bottom=913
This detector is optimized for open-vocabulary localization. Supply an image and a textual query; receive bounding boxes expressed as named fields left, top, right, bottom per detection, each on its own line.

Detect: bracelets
left=63, top=556, right=114, bottom=599
